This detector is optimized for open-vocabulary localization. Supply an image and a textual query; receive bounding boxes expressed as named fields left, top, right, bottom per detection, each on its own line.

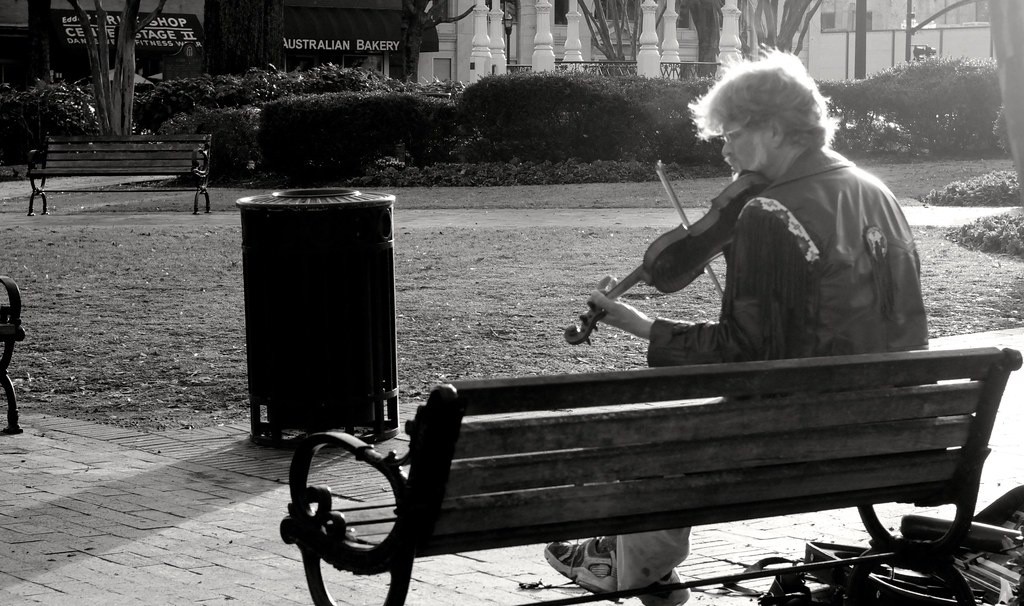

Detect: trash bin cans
left=233, top=187, right=400, bottom=450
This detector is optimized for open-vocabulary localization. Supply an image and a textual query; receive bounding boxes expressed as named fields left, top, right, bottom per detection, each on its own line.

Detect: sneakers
left=544, top=537, right=618, bottom=603
left=637, top=569, right=690, bottom=606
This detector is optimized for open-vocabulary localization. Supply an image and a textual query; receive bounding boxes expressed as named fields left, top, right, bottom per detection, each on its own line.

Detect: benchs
left=25, top=135, right=212, bottom=216
left=279, top=347, right=1024, bottom=606
left=0, top=274, right=25, bottom=434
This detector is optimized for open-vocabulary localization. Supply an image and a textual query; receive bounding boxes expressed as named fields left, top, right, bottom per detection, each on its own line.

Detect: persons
left=544, top=55, right=927, bottom=606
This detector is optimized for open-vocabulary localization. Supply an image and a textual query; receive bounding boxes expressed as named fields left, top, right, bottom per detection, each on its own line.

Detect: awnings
left=49, top=7, right=208, bottom=54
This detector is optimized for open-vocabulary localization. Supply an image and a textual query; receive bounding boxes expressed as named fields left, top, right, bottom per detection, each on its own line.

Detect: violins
left=563, top=172, right=771, bottom=346
